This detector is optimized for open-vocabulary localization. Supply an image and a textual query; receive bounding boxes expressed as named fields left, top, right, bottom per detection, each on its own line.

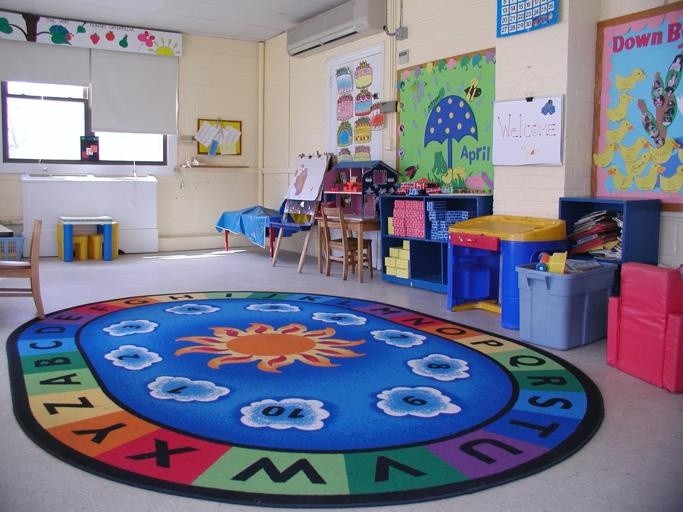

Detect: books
left=569, top=207, right=623, bottom=258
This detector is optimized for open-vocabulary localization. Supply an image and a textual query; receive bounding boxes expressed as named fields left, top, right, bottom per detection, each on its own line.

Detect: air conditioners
left=285, top=1, right=386, bottom=61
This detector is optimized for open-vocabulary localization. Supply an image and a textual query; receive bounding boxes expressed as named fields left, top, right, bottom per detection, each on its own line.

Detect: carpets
left=10, top=290, right=609, bottom=505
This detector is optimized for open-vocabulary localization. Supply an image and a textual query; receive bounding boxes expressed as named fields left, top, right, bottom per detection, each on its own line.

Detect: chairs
left=319, top=206, right=373, bottom=283
left=0, top=218, right=45, bottom=321
left=602, top=260, right=682, bottom=394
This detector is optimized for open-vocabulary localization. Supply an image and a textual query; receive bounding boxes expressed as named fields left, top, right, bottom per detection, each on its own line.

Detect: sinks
left=112, top=178, right=144, bottom=180
left=29, top=174, right=62, bottom=178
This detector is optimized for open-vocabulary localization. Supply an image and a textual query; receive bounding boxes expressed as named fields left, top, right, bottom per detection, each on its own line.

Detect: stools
left=58, top=215, right=113, bottom=263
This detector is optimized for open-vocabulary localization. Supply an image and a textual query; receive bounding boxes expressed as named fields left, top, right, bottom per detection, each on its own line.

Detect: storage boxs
left=514, top=257, right=616, bottom=352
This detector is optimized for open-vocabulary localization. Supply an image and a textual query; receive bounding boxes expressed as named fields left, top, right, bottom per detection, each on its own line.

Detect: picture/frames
left=197, top=119, right=242, bottom=156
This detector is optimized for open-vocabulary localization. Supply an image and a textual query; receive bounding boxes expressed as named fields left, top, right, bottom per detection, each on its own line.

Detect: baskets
left=0, top=235, right=24, bottom=261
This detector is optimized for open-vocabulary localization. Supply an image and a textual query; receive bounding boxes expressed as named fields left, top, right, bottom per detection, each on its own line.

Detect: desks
left=314, top=214, right=379, bottom=273
left=0, top=221, right=14, bottom=237
left=214, top=210, right=275, bottom=256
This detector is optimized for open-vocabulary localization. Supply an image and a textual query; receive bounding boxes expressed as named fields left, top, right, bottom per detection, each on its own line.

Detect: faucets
left=132, top=160, right=138, bottom=176
left=38, top=158, right=48, bottom=175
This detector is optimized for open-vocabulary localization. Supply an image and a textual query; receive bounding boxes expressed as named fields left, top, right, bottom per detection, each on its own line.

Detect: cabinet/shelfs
left=378, top=193, right=492, bottom=294
left=556, top=196, right=662, bottom=292
